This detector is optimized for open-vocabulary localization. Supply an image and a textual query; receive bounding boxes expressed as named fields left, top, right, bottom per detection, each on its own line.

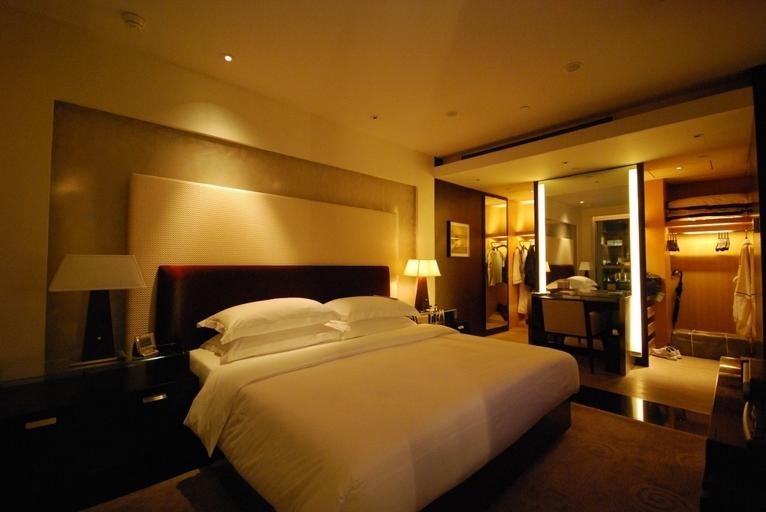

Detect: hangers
left=665, top=232, right=680, bottom=251
left=489, top=240, right=507, bottom=250
left=743, top=228, right=749, bottom=240
left=519, top=240, right=535, bottom=250
left=715, top=233, right=730, bottom=251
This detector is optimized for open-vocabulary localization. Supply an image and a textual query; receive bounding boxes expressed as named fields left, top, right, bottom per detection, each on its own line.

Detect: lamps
left=46, top=253, right=147, bottom=372
left=402, top=258, right=441, bottom=313
left=579, top=261, right=592, bottom=278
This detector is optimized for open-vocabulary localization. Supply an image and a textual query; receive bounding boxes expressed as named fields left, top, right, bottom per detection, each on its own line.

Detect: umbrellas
left=671, top=268, right=683, bottom=329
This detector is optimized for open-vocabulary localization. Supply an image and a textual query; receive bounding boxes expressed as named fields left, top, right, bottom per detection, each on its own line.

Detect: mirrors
left=540, top=166, right=632, bottom=298
left=480, top=191, right=510, bottom=337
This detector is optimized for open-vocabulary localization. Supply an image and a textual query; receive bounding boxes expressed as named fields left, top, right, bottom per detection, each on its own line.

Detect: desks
left=696, top=355, right=766, bottom=512
left=528, top=289, right=636, bottom=377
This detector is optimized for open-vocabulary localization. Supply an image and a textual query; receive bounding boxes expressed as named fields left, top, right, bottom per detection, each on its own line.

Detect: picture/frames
left=445, top=219, right=470, bottom=259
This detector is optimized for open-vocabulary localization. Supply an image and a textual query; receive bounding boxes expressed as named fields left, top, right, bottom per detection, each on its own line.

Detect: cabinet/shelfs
left=0, top=361, right=195, bottom=512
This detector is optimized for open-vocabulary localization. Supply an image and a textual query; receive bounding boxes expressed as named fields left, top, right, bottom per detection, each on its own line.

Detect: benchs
left=670, top=329, right=761, bottom=362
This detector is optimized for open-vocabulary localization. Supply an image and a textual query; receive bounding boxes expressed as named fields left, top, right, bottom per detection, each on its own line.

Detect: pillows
left=193, top=296, right=338, bottom=346
left=196, top=323, right=341, bottom=364
left=325, top=317, right=419, bottom=340
left=547, top=275, right=599, bottom=296
left=322, top=294, right=420, bottom=324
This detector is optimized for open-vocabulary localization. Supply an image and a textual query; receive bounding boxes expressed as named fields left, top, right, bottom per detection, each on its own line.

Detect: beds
left=546, top=264, right=631, bottom=297
left=156, top=263, right=582, bottom=511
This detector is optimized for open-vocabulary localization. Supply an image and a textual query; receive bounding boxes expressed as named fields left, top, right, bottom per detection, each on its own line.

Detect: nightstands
left=421, top=307, right=458, bottom=329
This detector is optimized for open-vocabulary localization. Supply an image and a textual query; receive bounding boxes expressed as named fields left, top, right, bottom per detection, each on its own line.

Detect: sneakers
left=651, top=345, right=682, bottom=361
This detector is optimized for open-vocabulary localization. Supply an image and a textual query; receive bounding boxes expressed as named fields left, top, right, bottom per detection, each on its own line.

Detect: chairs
left=537, top=296, right=620, bottom=375
